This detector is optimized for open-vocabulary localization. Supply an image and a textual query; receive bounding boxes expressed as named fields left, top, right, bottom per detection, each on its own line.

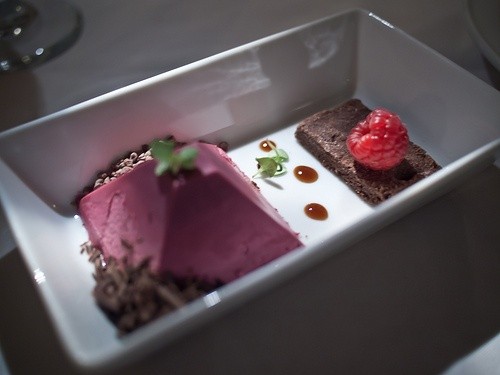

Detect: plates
left=463, top=1, right=500, bottom=69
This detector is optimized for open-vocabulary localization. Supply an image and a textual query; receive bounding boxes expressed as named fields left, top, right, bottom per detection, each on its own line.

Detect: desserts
left=76, top=97, right=443, bottom=336
